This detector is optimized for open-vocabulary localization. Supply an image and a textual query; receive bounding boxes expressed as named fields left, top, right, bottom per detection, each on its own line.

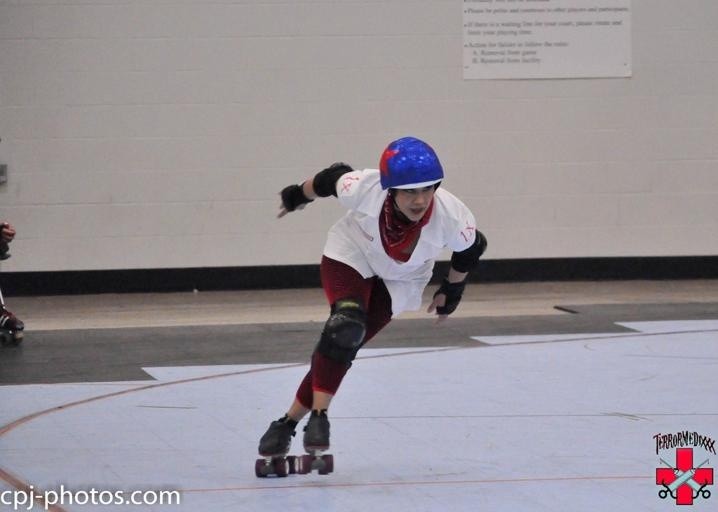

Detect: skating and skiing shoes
left=256, top=421, right=299, bottom=476
left=300, top=409, right=334, bottom=475
left=0, top=303, right=25, bottom=345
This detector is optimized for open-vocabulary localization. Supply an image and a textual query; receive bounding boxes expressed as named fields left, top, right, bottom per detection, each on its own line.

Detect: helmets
left=380, top=137, right=444, bottom=190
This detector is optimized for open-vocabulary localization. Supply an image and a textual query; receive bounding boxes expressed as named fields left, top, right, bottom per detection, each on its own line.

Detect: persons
left=252, top=136, right=486, bottom=456
left=0, top=217, right=24, bottom=348
